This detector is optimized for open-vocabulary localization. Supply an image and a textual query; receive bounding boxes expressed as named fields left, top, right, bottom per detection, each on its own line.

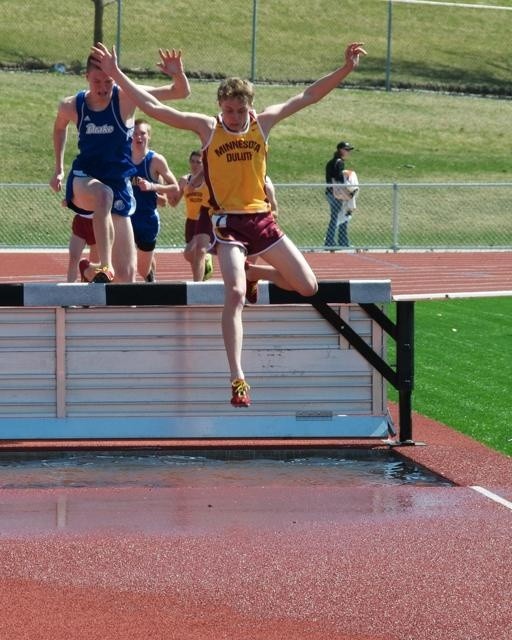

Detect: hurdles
left=0, top=279, right=429, bottom=447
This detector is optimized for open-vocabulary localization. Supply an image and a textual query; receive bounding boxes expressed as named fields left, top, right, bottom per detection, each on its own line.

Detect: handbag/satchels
left=331, top=176, right=355, bottom=203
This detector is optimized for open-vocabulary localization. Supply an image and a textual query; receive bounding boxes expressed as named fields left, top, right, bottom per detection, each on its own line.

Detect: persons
left=173, top=150, right=213, bottom=282
left=87, top=32, right=371, bottom=410
left=48, top=42, right=193, bottom=285
left=128, top=117, right=184, bottom=282
left=188, top=168, right=279, bottom=281
left=58, top=199, right=104, bottom=285
left=319, top=140, right=351, bottom=250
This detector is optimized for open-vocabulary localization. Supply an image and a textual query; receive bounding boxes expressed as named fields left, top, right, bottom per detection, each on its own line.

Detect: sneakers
left=231, top=378, right=251, bottom=408
left=79, top=259, right=91, bottom=282
left=245, top=260, right=260, bottom=305
left=203, top=253, right=213, bottom=281
left=144, top=257, right=156, bottom=282
left=93, top=266, right=116, bottom=284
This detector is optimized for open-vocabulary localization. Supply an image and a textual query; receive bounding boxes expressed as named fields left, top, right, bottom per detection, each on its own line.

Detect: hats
left=336, top=140, right=355, bottom=151
